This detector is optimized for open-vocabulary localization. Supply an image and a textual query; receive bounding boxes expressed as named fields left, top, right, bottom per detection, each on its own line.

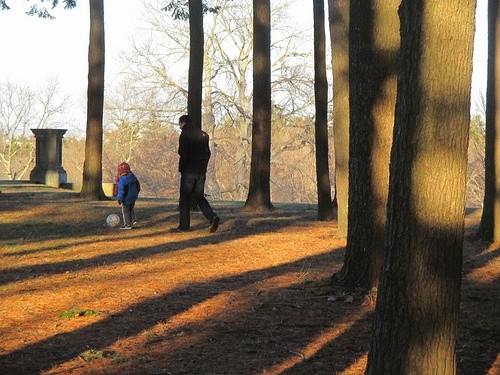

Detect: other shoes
left=133, top=222, right=137, bottom=226
left=118, top=225, right=131, bottom=229
left=171, top=226, right=191, bottom=232
left=210, top=216, right=219, bottom=233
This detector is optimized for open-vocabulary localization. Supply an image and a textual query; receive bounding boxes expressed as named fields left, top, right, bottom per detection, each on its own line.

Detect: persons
left=168, top=115, right=220, bottom=233
left=116, top=162, right=140, bottom=230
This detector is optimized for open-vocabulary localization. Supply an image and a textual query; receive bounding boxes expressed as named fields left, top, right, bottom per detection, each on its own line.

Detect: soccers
left=106, top=214, right=120, bottom=227
